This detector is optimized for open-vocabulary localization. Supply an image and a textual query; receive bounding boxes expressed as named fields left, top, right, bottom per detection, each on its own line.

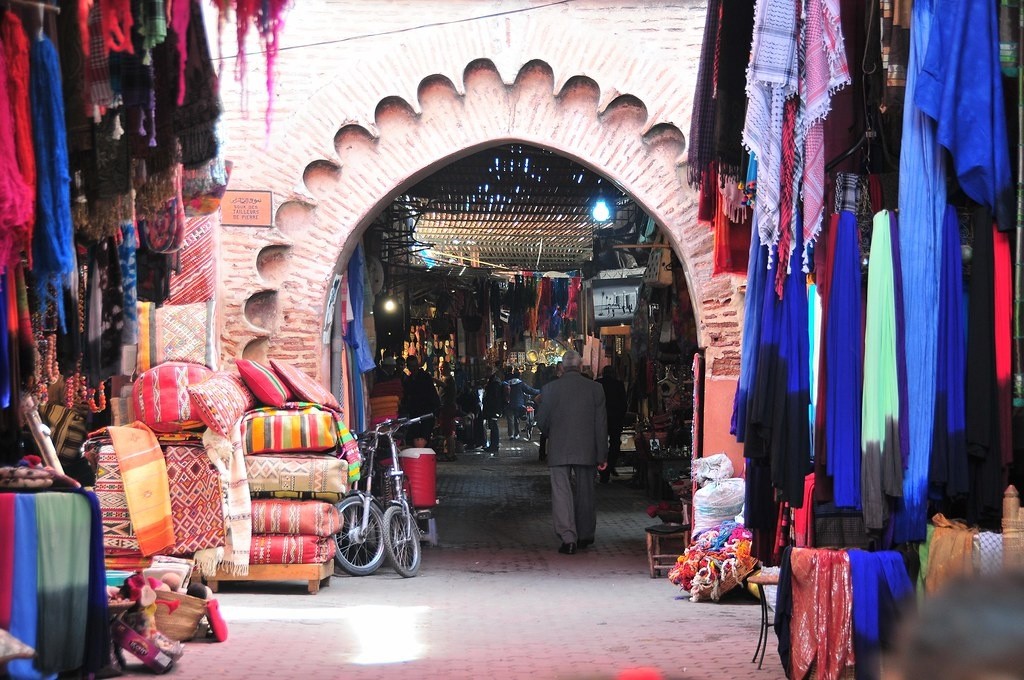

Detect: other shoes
left=509, top=434, right=521, bottom=440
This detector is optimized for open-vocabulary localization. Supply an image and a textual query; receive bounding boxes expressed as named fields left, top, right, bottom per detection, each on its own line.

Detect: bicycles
left=362, top=411, right=434, bottom=577
left=522, top=392, right=537, bottom=439
left=333, top=418, right=408, bottom=575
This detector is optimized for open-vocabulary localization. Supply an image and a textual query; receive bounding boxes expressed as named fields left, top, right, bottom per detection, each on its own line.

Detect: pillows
left=186, top=370, right=257, bottom=439
left=235, top=359, right=293, bottom=408
left=269, top=360, right=344, bottom=413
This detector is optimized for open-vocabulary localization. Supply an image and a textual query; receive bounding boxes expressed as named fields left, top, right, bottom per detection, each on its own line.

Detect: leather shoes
left=577, top=541, right=587, bottom=549
left=559, top=542, right=576, bottom=554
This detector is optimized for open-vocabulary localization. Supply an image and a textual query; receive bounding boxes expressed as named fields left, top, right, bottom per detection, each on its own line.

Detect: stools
left=747, top=577, right=779, bottom=669
left=646, top=524, right=691, bottom=579
left=409, top=507, right=440, bottom=548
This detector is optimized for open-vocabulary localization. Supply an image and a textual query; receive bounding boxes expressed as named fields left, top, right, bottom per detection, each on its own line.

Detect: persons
left=375, top=349, right=629, bottom=483
left=535, top=350, right=609, bottom=553
